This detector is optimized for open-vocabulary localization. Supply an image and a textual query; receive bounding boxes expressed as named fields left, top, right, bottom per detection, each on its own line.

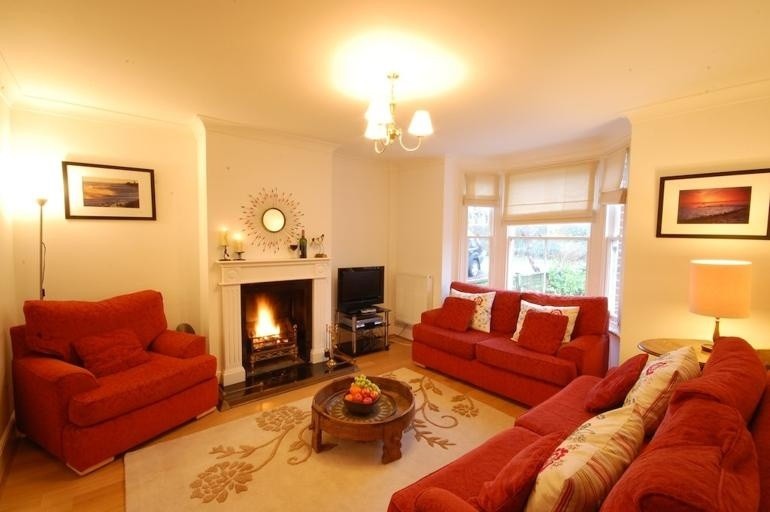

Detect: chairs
left=8, top=288, right=221, bottom=477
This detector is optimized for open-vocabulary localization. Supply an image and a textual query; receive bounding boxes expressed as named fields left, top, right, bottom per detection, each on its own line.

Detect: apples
left=344, top=385, right=377, bottom=404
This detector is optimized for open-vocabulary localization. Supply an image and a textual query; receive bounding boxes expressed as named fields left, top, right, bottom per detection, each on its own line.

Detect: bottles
left=299, top=229, right=307, bottom=258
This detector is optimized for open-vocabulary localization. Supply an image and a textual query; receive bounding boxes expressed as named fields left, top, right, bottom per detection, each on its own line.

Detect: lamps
left=33, top=198, right=49, bottom=300
left=363, top=65, right=435, bottom=154
left=686, top=258, right=757, bottom=354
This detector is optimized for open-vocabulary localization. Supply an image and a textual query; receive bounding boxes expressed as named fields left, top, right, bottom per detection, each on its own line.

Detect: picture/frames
left=62, top=159, right=158, bottom=221
left=653, top=167, right=770, bottom=241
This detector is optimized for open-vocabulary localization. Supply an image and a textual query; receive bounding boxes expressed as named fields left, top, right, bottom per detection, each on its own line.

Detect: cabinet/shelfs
left=336, top=305, right=392, bottom=357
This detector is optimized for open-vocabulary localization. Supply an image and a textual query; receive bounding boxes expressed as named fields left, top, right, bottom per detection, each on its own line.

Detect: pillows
left=448, top=287, right=498, bottom=334
left=467, top=426, right=570, bottom=511
left=516, top=308, right=570, bottom=359
left=624, top=344, right=702, bottom=434
left=436, top=297, right=478, bottom=333
left=584, top=352, right=650, bottom=412
left=524, top=407, right=646, bottom=510
left=509, top=298, right=583, bottom=356
left=72, top=327, right=149, bottom=378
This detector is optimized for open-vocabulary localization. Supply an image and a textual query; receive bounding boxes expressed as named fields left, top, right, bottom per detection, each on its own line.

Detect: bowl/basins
left=341, top=390, right=381, bottom=416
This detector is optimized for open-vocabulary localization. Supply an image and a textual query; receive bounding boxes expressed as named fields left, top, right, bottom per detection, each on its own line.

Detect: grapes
left=353, top=375, right=380, bottom=390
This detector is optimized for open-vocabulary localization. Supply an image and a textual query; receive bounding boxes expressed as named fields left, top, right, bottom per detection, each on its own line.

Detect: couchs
left=409, top=280, right=610, bottom=408
left=387, top=336, right=770, bottom=512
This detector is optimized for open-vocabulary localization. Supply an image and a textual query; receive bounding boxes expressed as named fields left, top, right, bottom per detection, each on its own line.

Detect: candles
left=232, top=234, right=243, bottom=253
left=218, top=224, right=229, bottom=246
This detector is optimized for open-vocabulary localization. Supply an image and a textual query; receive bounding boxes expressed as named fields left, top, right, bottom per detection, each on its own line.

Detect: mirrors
left=262, top=208, right=285, bottom=233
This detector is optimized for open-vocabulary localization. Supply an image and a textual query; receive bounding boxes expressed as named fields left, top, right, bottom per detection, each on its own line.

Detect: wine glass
left=289, top=239, right=298, bottom=259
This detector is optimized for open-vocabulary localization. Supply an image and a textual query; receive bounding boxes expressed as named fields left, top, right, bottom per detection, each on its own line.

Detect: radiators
left=393, top=272, right=432, bottom=325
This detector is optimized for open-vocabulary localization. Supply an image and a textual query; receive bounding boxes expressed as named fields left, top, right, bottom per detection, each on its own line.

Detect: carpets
left=121, top=367, right=517, bottom=512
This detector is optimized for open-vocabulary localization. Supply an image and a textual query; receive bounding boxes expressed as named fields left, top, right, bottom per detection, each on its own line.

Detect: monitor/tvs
left=337, top=266, right=384, bottom=312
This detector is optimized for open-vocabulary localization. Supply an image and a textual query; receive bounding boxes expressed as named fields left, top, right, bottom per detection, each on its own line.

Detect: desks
left=635, top=337, right=770, bottom=372
